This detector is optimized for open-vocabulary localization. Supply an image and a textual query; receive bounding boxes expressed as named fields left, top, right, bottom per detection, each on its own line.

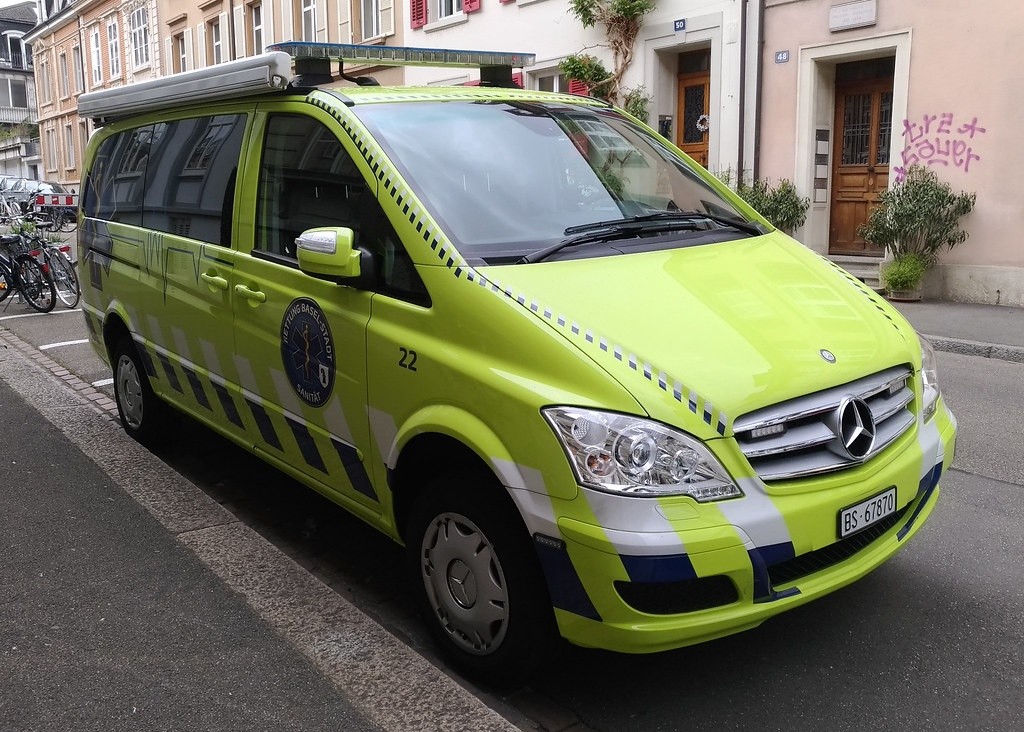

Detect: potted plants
left=855, top=163, right=976, bottom=300
left=0, top=116, right=37, bottom=150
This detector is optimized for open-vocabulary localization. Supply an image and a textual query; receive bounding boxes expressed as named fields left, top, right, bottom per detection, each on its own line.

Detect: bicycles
left=1, top=174, right=81, bottom=316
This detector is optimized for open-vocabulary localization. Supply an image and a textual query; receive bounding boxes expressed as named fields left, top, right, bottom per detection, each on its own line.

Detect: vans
left=69, top=37, right=961, bottom=685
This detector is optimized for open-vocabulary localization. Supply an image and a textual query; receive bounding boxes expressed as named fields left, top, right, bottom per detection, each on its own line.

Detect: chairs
left=280, top=173, right=361, bottom=258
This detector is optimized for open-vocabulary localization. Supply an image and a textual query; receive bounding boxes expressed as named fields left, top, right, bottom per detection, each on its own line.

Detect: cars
left=7, top=180, right=78, bottom=226
